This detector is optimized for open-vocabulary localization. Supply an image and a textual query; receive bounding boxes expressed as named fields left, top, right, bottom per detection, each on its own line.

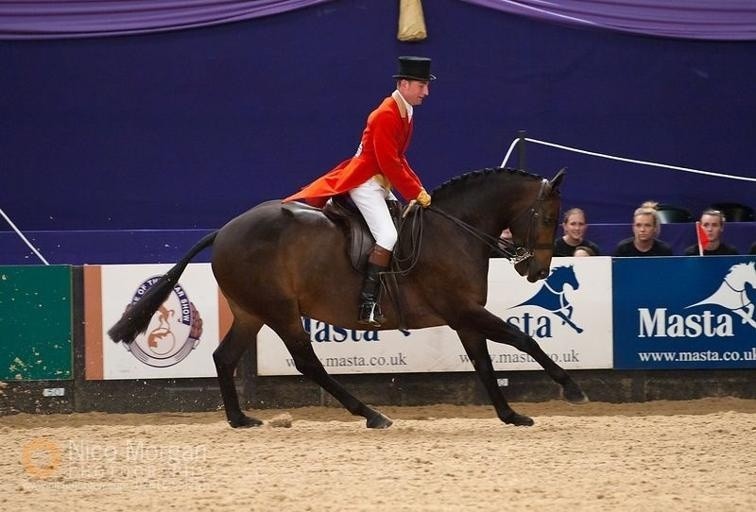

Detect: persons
left=282, top=54, right=437, bottom=326
left=551, top=206, right=608, bottom=256
left=683, top=208, right=742, bottom=255
left=612, top=200, right=673, bottom=256
left=489, top=228, right=516, bottom=258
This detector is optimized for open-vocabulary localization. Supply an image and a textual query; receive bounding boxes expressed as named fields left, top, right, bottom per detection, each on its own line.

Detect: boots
left=358, top=262, right=393, bottom=325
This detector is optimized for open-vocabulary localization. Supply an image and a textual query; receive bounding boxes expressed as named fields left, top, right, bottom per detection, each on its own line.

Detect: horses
left=107, top=165, right=591, bottom=430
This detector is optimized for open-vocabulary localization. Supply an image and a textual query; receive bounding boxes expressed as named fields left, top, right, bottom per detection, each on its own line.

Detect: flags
left=696, top=223, right=709, bottom=251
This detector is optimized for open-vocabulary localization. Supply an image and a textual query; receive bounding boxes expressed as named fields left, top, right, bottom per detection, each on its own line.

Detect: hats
left=392, top=56, right=436, bottom=81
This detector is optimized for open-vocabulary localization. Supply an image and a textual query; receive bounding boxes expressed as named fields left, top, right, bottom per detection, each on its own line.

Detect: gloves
left=418, top=187, right=431, bottom=207
